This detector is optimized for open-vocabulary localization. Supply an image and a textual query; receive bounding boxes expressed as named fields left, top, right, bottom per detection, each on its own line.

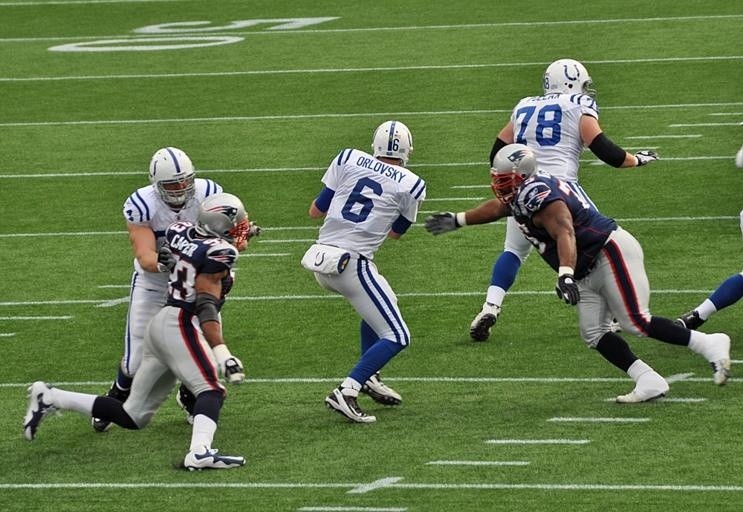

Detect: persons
left=470, top=58, right=659, bottom=342
left=91, top=147, right=260, bottom=432
left=425, top=143, right=731, bottom=404
left=20, top=193, right=246, bottom=472
left=673, top=146, right=743, bottom=329
left=301, top=121, right=425, bottom=423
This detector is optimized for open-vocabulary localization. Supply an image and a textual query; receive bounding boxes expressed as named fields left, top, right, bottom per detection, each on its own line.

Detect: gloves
left=220, top=354, right=245, bottom=384
left=424, top=211, right=466, bottom=235
left=635, top=151, right=660, bottom=166
left=555, top=265, right=580, bottom=305
left=157, top=246, right=176, bottom=273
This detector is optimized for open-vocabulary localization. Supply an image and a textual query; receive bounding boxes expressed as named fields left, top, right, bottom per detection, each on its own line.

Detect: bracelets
left=633, top=156, right=639, bottom=166
left=454, top=212, right=467, bottom=228
left=209, top=345, right=230, bottom=362
left=558, top=266, right=575, bottom=278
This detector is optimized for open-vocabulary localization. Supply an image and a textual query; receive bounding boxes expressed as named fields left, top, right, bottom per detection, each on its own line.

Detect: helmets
left=371, top=121, right=413, bottom=166
left=149, top=147, right=196, bottom=205
left=543, top=58, right=597, bottom=97
left=195, top=193, right=250, bottom=251
left=489, top=143, right=536, bottom=207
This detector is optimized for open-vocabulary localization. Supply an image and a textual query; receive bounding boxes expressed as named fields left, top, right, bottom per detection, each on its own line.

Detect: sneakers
left=705, top=332, right=732, bottom=386
left=23, top=379, right=56, bottom=441
left=176, top=383, right=198, bottom=425
left=184, top=444, right=246, bottom=471
left=469, top=302, right=502, bottom=341
left=91, top=387, right=130, bottom=431
left=615, top=376, right=669, bottom=403
left=674, top=310, right=707, bottom=330
left=324, top=386, right=376, bottom=423
left=360, top=371, right=402, bottom=405
